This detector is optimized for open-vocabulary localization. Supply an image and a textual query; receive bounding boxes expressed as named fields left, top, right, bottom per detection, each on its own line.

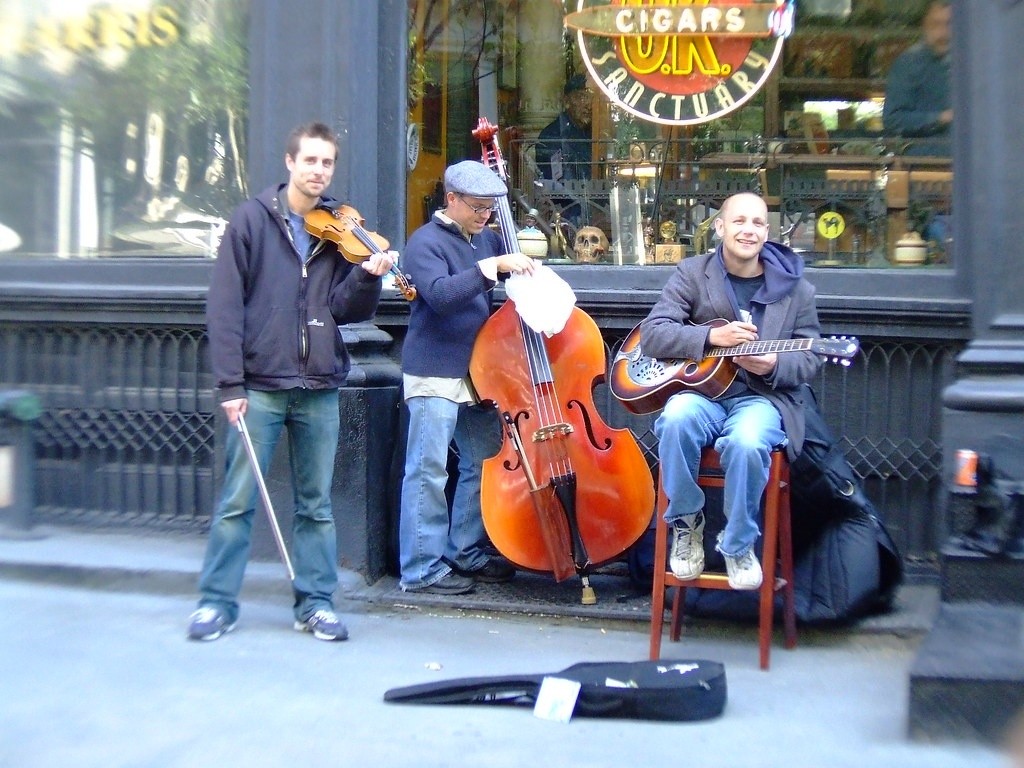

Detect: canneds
left=956, top=449, right=978, bottom=487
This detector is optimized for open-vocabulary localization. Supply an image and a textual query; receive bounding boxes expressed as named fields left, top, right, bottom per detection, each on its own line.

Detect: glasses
left=454, top=192, right=497, bottom=214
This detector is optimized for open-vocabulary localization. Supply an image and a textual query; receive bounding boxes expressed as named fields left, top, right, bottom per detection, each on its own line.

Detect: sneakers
left=670, top=512, right=705, bottom=582
left=724, top=545, right=762, bottom=590
left=408, top=570, right=476, bottom=594
left=453, top=555, right=516, bottom=582
left=294, top=609, right=350, bottom=640
left=187, top=606, right=238, bottom=642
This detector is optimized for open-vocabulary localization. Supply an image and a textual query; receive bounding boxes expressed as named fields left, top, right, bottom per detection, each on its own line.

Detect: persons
left=397, top=161, right=539, bottom=593
left=535, top=75, right=611, bottom=233
left=640, top=193, right=823, bottom=588
left=885, top=1, right=959, bottom=253
left=188, top=121, right=395, bottom=641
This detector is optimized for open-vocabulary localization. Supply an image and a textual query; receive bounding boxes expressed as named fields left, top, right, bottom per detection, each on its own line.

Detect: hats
left=444, top=160, right=508, bottom=199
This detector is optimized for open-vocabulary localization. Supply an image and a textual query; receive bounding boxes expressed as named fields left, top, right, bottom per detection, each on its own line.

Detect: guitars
left=609, top=315, right=862, bottom=417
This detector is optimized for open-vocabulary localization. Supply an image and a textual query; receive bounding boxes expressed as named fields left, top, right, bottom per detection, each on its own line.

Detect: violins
left=303, top=203, right=418, bottom=302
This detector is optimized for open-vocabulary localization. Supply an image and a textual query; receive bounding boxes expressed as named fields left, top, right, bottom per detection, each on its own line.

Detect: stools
left=648, top=437, right=796, bottom=670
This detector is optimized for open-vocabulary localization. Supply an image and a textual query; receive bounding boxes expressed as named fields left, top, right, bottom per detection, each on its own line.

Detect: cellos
left=470, top=114, right=657, bottom=603
